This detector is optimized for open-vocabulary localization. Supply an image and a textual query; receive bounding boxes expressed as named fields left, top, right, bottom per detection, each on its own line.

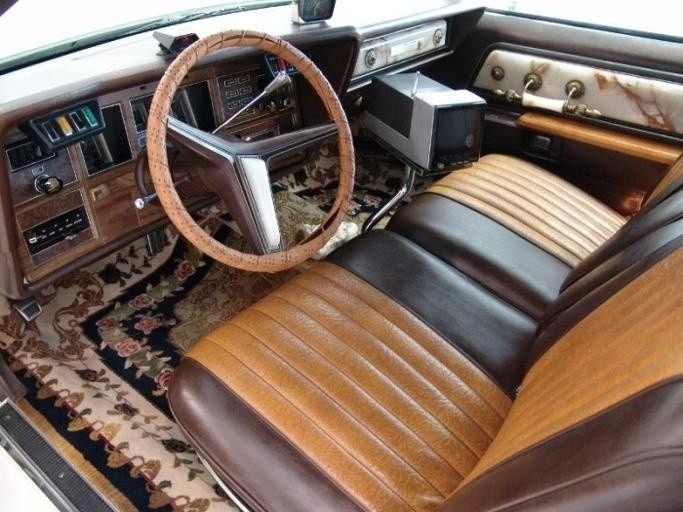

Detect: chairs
left=384, top=151, right=682, bottom=319
left=165, top=227, right=682, bottom=512
